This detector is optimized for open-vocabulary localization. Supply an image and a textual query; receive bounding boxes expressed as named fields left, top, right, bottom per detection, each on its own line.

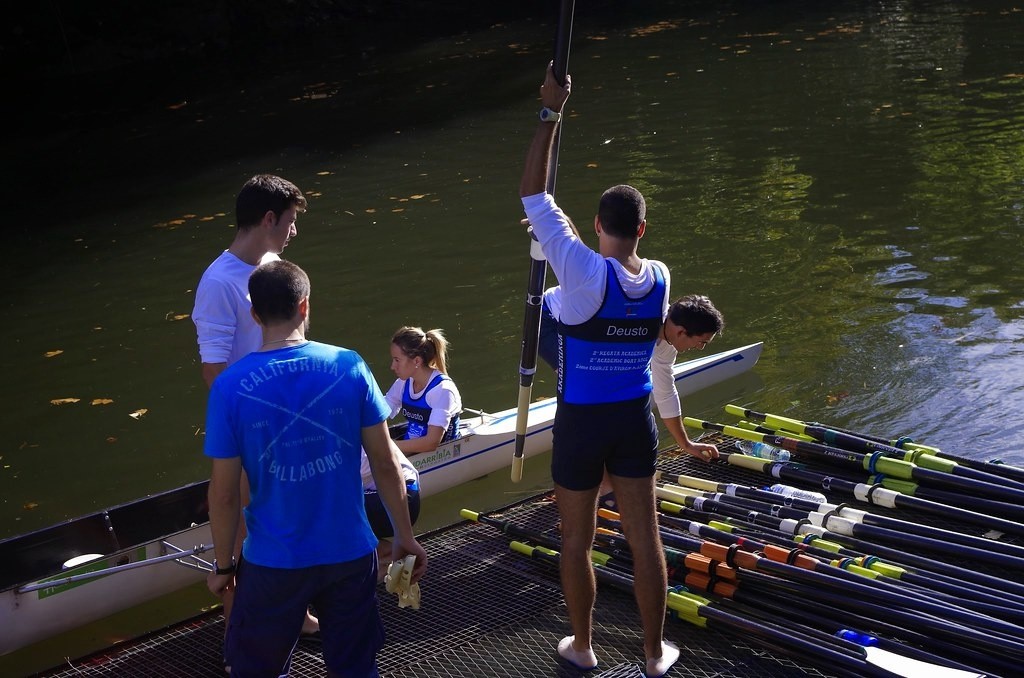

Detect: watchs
left=540, top=107, right=560, bottom=125
left=212, top=558, right=237, bottom=575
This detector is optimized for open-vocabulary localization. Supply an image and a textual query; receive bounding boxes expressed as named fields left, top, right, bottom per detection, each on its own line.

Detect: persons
left=192, top=173, right=324, bottom=635
left=539, top=285, right=723, bottom=463
left=356, top=425, right=420, bottom=586
left=519, top=57, right=681, bottom=676
left=381, top=322, right=461, bottom=459
left=205, top=261, right=426, bottom=678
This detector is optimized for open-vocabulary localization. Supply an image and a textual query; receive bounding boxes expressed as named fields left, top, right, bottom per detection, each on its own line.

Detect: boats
left=1, top=340, right=767, bottom=654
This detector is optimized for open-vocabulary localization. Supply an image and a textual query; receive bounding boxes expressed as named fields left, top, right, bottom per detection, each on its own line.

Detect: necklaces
left=262, top=339, right=305, bottom=348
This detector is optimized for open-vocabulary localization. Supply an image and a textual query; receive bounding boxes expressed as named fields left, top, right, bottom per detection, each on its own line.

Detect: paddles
left=459, top=0, right=1024, bottom=678
